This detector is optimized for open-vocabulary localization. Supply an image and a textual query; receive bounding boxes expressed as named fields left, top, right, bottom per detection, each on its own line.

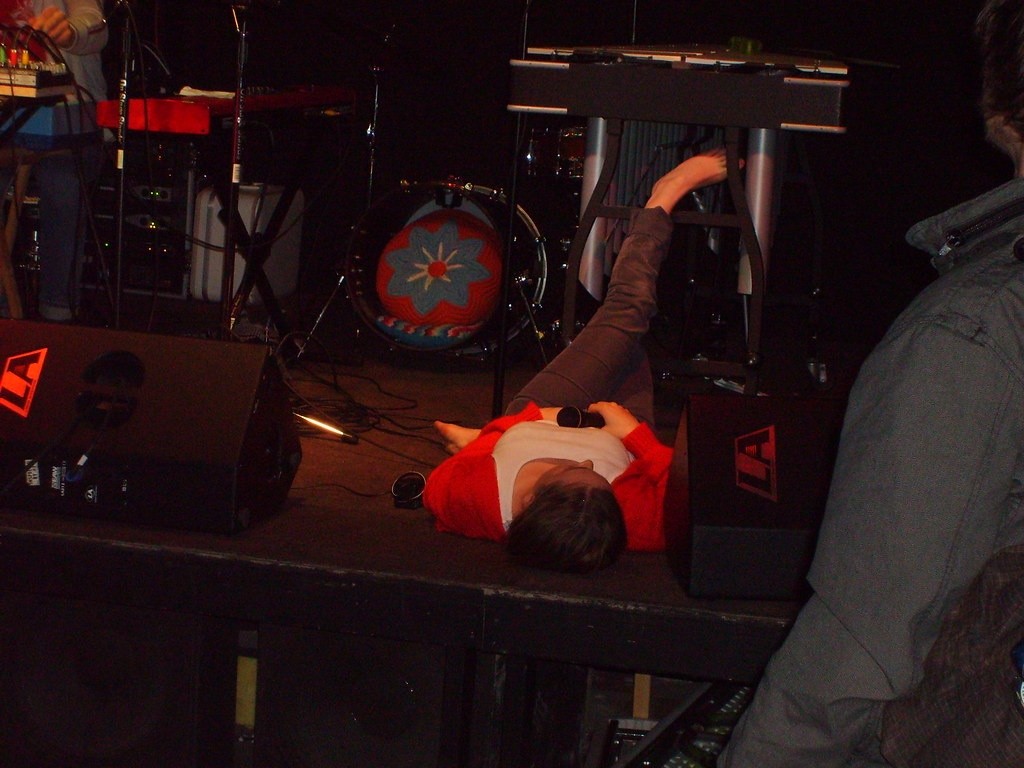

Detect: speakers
left=0, top=319, right=302, bottom=534
left=661, top=391, right=848, bottom=601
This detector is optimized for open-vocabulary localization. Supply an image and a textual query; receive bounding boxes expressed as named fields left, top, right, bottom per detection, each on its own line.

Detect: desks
left=504, top=61, right=850, bottom=395
left=96, top=84, right=374, bottom=371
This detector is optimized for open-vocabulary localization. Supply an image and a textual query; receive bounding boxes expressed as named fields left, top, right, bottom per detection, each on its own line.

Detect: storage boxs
left=191, top=182, right=304, bottom=304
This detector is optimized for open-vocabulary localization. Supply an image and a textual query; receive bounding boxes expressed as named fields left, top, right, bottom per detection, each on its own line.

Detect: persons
left=0, top=0, right=109, bottom=320
left=420, top=145, right=746, bottom=575
left=714, top=0, right=1024, bottom=768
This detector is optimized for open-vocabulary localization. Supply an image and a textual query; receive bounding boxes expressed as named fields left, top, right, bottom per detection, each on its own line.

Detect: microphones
left=557, top=405, right=606, bottom=430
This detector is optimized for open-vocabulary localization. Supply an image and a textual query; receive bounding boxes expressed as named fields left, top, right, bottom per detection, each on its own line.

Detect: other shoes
left=37, top=292, right=72, bottom=321
